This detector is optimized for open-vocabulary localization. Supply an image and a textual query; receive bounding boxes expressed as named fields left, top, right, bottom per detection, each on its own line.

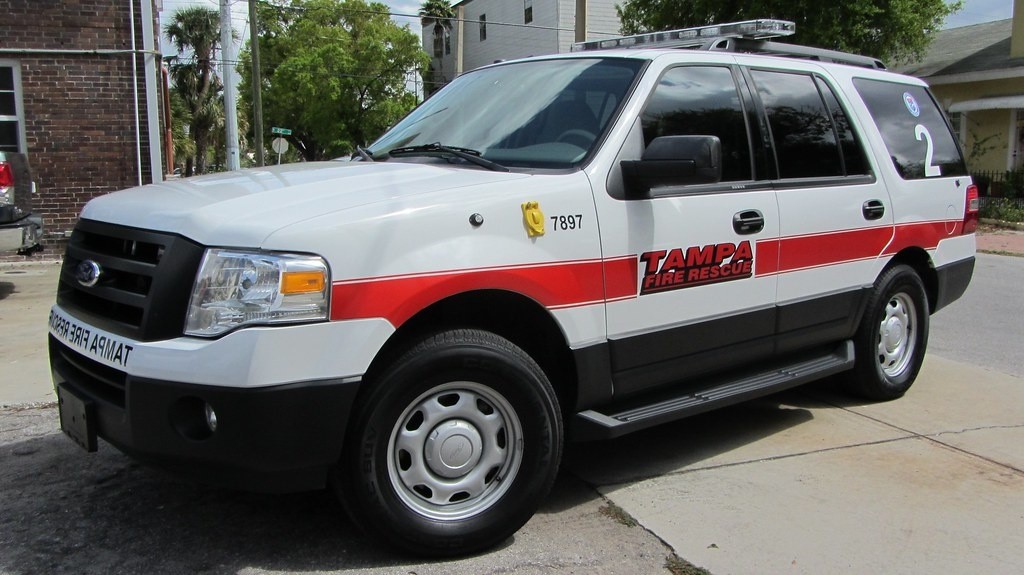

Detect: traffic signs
left=272, top=128, right=291, bottom=135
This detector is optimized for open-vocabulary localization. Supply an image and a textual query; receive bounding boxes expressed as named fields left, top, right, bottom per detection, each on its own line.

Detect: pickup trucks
left=0, top=150, right=42, bottom=256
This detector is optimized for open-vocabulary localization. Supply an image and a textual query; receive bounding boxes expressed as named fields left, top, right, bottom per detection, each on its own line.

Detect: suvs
left=42, top=19, right=979, bottom=557
